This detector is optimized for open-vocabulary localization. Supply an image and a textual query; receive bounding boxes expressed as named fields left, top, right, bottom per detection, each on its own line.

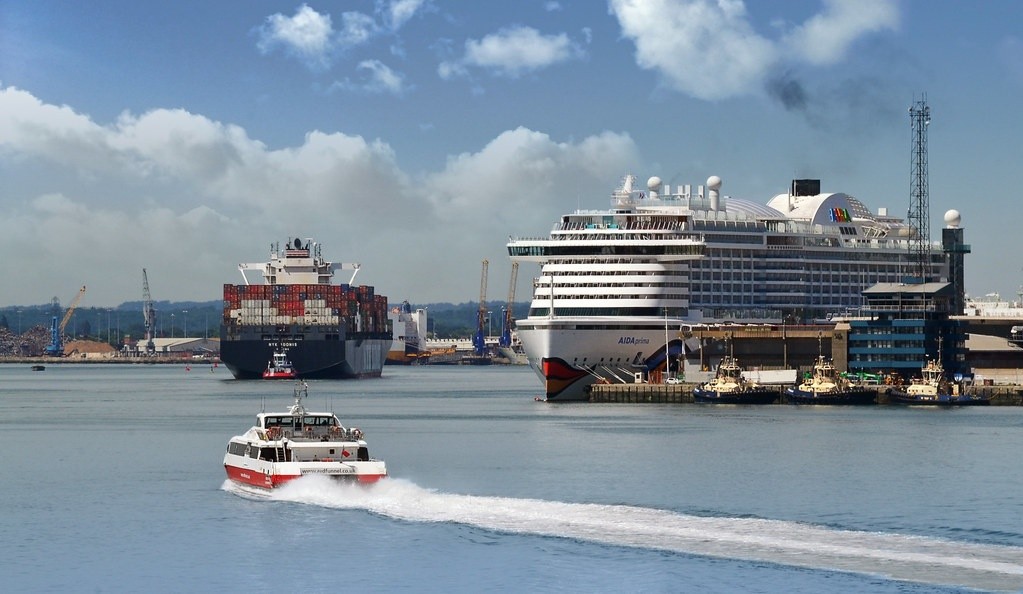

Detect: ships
left=219, top=236, right=393, bottom=381
left=506, top=90, right=1023, bottom=403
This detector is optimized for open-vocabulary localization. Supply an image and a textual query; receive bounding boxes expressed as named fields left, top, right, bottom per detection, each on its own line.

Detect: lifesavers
left=353, top=429, right=360, bottom=435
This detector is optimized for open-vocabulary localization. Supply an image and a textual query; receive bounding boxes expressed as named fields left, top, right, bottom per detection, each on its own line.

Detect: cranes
left=46, top=283, right=87, bottom=358
left=495, top=260, right=519, bottom=359
left=472, top=257, right=491, bottom=358
left=141, top=268, right=158, bottom=358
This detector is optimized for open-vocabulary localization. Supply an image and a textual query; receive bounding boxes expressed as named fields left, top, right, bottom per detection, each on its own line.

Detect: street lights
left=17, top=311, right=23, bottom=336
left=96, top=312, right=102, bottom=342
left=183, top=310, right=189, bottom=338
left=171, top=313, right=176, bottom=338
left=106, top=309, right=111, bottom=344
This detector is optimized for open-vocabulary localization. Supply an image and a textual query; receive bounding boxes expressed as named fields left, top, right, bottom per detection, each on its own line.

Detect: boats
left=691, top=329, right=781, bottom=404
left=884, top=328, right=990, bottom=406
left=222, top=378, right=386, bottom=491
left=31, top=365, right=45, bottom=371
left=781, top=330, right=879, bottom=406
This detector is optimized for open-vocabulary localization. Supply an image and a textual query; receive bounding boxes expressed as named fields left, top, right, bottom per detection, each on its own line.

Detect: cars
left=665, top=378, right=683, bottom=384
